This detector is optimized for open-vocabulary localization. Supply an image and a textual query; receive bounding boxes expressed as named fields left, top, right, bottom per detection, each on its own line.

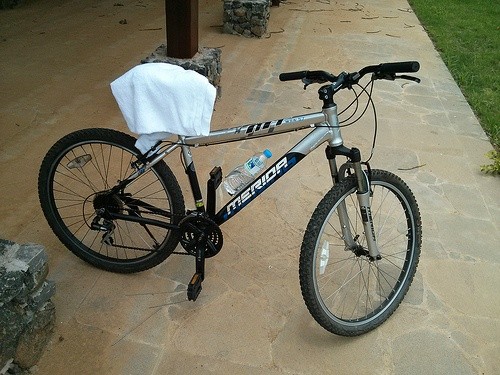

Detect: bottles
left=224, top=149, right=272, bottom=195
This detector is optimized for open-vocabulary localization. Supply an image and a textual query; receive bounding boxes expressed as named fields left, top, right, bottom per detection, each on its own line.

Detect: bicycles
left=37, top=60, right=423, bottom=337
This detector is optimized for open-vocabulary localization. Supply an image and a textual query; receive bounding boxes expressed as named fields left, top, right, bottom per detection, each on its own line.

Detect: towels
left=110, top=63, right=217, bottom=158
